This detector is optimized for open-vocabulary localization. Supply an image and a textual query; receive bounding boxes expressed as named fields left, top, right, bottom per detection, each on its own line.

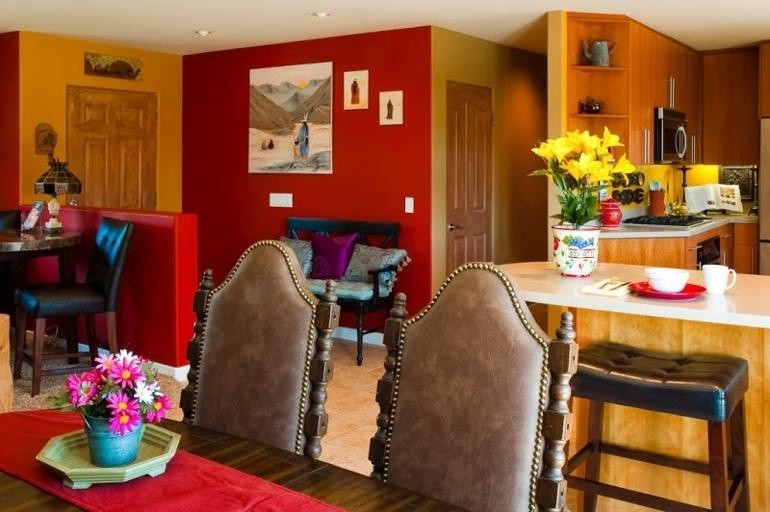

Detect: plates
left=629, top=283, right=707, bottom=300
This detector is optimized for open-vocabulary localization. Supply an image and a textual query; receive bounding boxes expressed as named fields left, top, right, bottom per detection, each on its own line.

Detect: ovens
left=696, top=236, right=721, bottom=271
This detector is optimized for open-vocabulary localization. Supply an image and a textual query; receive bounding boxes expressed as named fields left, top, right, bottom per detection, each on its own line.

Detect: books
left=684, top=184, right=744, bottom=214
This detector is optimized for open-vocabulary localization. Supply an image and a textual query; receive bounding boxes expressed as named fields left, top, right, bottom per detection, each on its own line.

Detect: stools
left=561, top=341, right=750, bottom=512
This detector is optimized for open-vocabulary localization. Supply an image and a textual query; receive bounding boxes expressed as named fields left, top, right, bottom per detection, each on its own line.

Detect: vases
left=79, top=406, right=147, bottom=468
left=551, top=224, right=602, bottom=279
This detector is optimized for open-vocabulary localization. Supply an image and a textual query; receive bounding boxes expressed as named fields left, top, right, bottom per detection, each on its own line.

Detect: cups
left=702, top=264, right=737, bottom=297
left=649, top=191, right=665, bottom=218
left=645, top=267, right=690, bottom=292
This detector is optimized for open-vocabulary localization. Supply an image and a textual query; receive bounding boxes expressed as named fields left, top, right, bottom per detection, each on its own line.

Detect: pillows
left=279, top=229, right=412, bottom=287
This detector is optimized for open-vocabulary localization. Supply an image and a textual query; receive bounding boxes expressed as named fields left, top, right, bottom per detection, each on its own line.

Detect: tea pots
left=581, top=39, right=617, bottom=67
left=580, top=98, right=605, bottom=113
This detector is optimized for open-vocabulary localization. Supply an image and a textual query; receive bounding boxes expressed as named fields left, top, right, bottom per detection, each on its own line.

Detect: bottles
left=600, top=200, right=623, bottom=228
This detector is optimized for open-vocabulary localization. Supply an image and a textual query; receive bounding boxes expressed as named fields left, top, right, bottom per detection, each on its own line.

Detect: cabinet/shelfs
left=564, top=11, right=759, bottom=166
left=721, top=224, right=733, bottom=271
left=733, top=220, right=760, bottom=275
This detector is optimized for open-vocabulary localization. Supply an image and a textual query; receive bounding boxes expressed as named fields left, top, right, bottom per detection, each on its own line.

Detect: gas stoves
left=625, top=214, right=712, bottom=229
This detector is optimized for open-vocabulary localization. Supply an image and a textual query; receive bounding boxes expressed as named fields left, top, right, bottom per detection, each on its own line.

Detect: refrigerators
left=759, top=117, right=770, bottom=274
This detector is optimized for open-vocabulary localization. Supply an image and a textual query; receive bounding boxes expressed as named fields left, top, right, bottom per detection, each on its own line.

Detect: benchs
left=279, top=217, right=411, bottom=365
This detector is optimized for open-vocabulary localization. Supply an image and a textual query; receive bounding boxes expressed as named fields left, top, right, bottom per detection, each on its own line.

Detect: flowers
left=65, top=348, right=174, bottom=437
left=526, top=125, right=637, bottom=230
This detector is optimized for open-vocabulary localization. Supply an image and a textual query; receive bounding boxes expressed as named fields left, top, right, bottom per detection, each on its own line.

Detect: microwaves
left=654, top=107, right=689, bottom=164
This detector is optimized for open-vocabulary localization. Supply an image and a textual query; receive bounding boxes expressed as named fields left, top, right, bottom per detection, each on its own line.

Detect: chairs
left=13, top=216, right=136, bottom=398
left=0, top=210, right=23, bottom=316
left=179, top=240, right=341, bottom=460
left=368, top=260, right=580, bottom=512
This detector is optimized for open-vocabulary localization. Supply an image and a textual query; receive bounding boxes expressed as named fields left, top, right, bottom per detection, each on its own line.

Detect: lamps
left=32, top=159, right=81, bottom=200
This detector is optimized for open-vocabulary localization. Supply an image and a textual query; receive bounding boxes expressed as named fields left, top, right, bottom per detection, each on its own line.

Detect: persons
left=295, top=119, right=310, bottom=161
left=293, top=140, right=300, bottom=161
